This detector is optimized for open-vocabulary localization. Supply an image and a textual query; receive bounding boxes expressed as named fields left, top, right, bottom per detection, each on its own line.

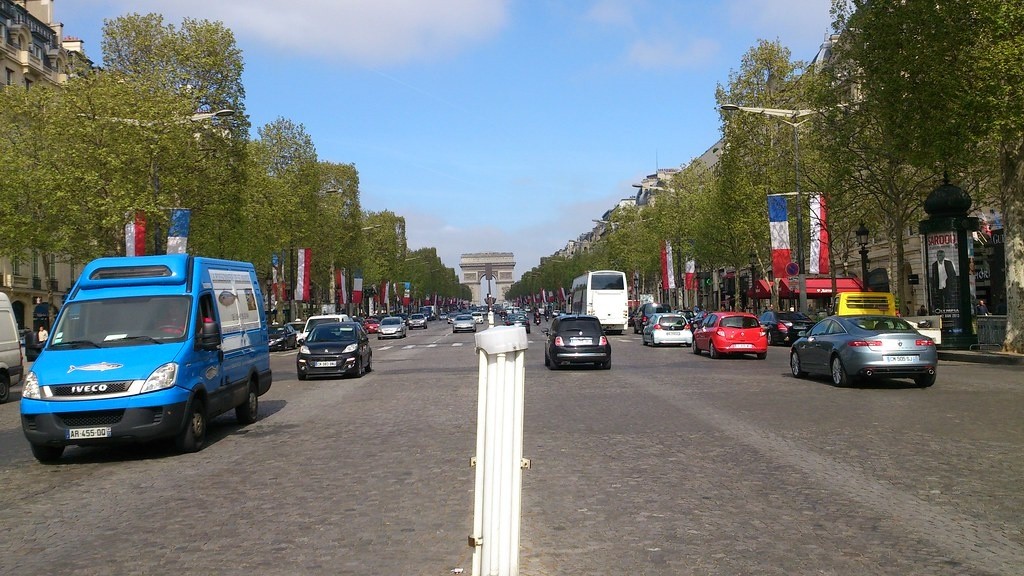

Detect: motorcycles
left=545, top=315, right=549, bottom=322
left=534, top=313, right=541, bottom=326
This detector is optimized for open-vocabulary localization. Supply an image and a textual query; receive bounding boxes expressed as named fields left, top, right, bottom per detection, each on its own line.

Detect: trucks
left=827, top=291, right=942, bottom=344
left=20, top=253, right=272, bottom=462
left=0, top=291, right=23, bottom=403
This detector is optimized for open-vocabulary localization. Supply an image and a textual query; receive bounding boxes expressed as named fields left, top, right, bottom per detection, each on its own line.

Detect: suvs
left=300, top=315, right=350, bottom=341
left=552, top=310, right=560, bottom=318
left=408, top=314, right=428, bottom=330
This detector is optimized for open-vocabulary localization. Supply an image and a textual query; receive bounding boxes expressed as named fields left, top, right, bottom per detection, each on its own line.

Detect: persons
left=823, top=303, right=828, bottom=309
left=808, top=304, right=813, bottom=311
left=534, top=309, right=541, bottom=324
left=977, top=300, right=990, bottom=316
left=694, top=307, right=698, bottom=316
left=39, top=325, right=48, bottom=343
left=721, top=305, right=726, bottom=311
left=917, top=305, right=928, bottom=316
left=906, top=300, right=912, bottom=315
left=629, top=307, right=633, bottom=317
left=826, top=305, right=832, bottom=316
left=932, top=250, right=956, bottom=310
left=154, top=299, right=186, bottom=334
left=544, top=309, right=549, bottom=317
left=763, top=307, right=768, bottom=313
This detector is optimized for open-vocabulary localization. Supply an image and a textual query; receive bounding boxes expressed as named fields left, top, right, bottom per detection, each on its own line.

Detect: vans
left=632, top=303, right=672, bottom=334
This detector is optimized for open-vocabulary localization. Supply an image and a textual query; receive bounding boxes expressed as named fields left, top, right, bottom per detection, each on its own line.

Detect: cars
left=352, top=313, right=409, bottom=334
left=377, top=317, right=406, bottom=340
left=268, top=318, right=306, bottom=352
left=296, top=322, right=373, bottom=380
left=692, top=312, right=768, bottom=359
left=438, top=308, right=484, bottom=333
left=452, top=314, right=476, bottom=333
left=642, top=313, right=693, bottom=347
left=629, top=309, right=695, bottom=325
left=495, top=307, right=530, bottom=333
left=759, top=311, right=816, bottom=346
left=420, top=308, right=436, bottom=321
left=789, top=315, right=938, bottom=387
left=541, top=315, right=612, bottom=370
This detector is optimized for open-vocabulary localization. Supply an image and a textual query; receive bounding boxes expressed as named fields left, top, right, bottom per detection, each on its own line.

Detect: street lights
left=855, top=219, right=870, bottom=290
left=721, top=104, right=880, bottom=314
left=77, top=109, right=237, bottom=252
left=634, top=276, right=639, bottom=301
left=748, top=246, right=757, bottom=310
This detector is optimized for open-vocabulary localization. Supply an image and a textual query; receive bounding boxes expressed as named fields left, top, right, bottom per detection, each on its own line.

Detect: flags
left=980, top=210, right=992, bottom=239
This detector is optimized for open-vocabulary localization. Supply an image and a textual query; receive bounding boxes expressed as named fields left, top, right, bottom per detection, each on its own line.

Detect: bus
left=505, top=307, right=520, bottom=314
left=568, top=270, right=629, bottom=335
